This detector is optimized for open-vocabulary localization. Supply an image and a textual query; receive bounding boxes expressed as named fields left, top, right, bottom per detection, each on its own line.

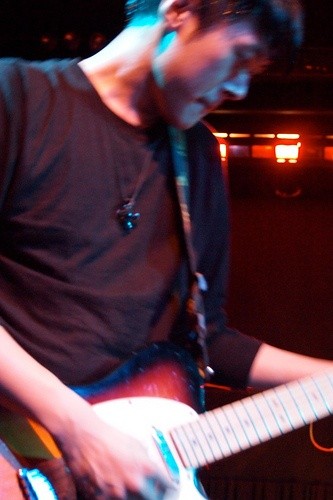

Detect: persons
left=0, top=0, right=333, bottom=500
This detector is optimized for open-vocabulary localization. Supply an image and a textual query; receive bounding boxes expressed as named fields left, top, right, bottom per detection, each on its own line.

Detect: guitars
left=0, top=343, right=332, bottom=500
